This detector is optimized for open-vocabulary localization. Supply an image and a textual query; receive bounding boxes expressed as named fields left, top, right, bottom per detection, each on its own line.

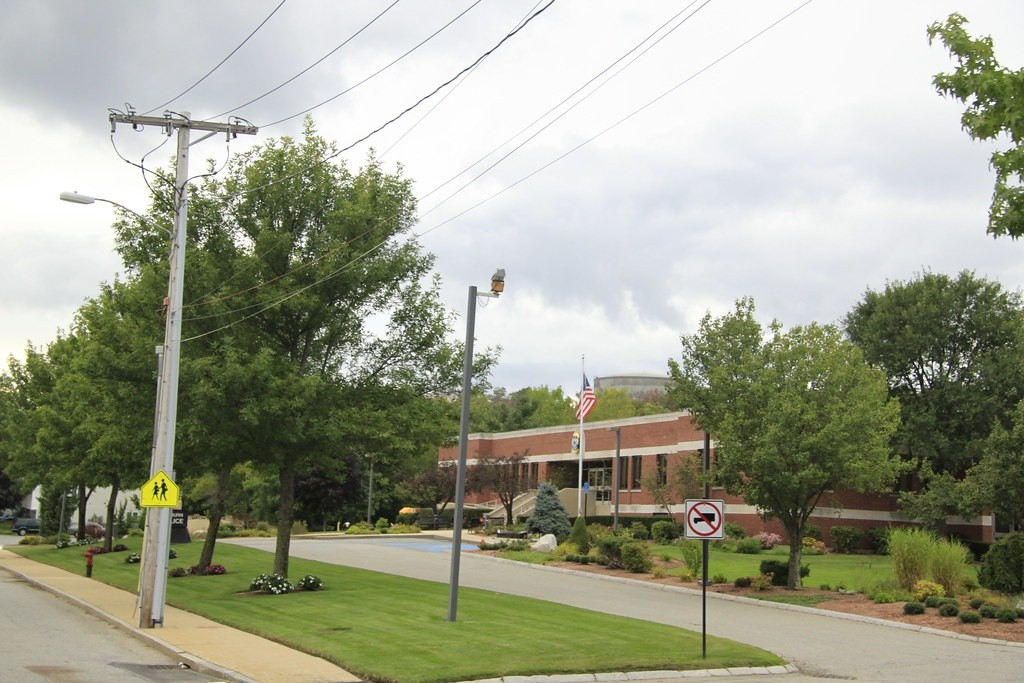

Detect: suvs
left=11, top=517, right=41, bottom=536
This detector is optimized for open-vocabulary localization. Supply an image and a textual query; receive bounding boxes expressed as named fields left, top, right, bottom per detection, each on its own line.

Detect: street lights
left=58, top=190, right=183, bottom=626
left=606, top=426, right=619, bottom=534
left=365, top=453, right=373, bottom=522
left=448, top=266, right=506, bottom=622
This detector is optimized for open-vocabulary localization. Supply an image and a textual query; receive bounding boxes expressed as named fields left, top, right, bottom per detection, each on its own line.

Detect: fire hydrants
left=82, top=547, right=93, bottom=578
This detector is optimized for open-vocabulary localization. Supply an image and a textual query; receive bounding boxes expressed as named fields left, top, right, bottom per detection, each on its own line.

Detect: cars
left=68, top=522, right=106, bottom=538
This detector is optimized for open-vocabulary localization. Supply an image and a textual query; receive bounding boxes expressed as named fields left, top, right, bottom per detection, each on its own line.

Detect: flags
left=576, top=376, right=596, bottom=420
